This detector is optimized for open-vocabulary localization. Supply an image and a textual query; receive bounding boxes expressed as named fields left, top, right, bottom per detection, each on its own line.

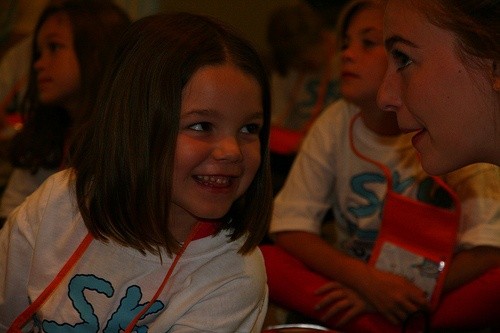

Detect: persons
left=257, top=0, right=500, bottom=333
left=0, top=13, right=272, bottom=333
left=377, top=0, right=500, bottom=177
left=0, top=0, right=131, bottom=229
